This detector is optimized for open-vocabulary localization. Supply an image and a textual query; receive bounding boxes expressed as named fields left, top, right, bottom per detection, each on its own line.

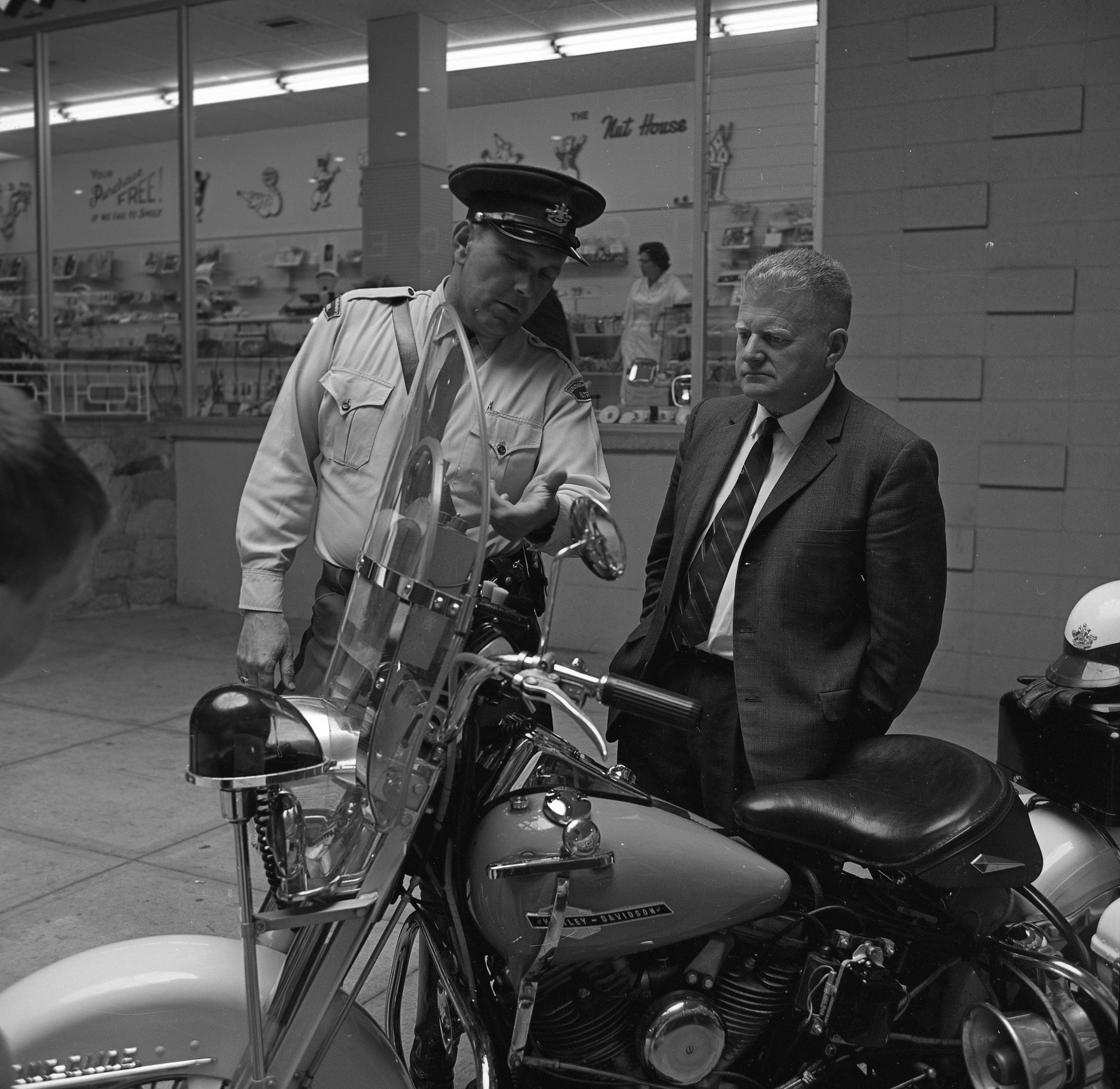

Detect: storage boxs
left=996, top=690, right=1120, bottom=825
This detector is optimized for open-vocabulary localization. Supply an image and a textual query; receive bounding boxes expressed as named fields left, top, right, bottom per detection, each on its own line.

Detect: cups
left=633, top=409, right=651, bottom=424
left=657, top=406, right=678, bottom=421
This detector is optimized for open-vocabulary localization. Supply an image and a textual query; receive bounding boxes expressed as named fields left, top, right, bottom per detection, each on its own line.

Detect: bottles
left=610, top=240, right=626, bottom=261
left=581, top=240, right=598, bottom=262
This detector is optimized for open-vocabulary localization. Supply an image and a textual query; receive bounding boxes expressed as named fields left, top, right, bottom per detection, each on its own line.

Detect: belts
left=660, top=640, right=734, bottom=670
left=322, top=563, right=354, bottom=594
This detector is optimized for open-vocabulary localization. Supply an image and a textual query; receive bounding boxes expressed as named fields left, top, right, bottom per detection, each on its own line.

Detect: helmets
left=1046, top=580, right=1120, bottom=688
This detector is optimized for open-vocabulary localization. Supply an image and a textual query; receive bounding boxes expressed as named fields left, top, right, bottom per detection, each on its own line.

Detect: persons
left=610, top=239, right=693, bottom=407
left=0, top=379, right=110, bottom=674
left=235, top=162, right=614, bottom=841
left=595, top=247, right=951, bottom=854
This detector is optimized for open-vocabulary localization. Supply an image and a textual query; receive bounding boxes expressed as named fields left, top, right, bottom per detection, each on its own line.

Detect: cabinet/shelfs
left=193, top=261, right=358, bottom=362
left=556, top=254, right=623, bottom=376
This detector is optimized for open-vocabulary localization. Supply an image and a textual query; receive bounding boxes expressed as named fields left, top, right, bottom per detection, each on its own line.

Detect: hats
left=449, top=163, right=606, bottom=266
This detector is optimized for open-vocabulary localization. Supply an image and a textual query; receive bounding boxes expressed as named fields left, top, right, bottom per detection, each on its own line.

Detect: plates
left=655, top=420, right=675, bottom=424
left=597, top=404, right=620, bottom=423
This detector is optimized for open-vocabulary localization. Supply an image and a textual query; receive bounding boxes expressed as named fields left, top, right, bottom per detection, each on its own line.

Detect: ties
left=423, top=330, right=473, bottom=441
left=669, top=417, right=781, bottom=648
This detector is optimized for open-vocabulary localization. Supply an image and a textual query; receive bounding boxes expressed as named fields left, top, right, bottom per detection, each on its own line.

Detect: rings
left=239, top=676, right=249, bottom=685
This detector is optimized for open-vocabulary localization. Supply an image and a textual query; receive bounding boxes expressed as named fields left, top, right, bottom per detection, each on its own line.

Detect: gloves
left=1014, top=676, right=1096, bottom=720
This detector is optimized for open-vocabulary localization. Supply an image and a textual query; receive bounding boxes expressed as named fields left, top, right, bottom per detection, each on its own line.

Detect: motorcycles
left=1, top=302, right=1120, bottom=1089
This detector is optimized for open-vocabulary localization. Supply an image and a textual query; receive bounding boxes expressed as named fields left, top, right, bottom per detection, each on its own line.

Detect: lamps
left=0, top=1, right=818, bottom=134
